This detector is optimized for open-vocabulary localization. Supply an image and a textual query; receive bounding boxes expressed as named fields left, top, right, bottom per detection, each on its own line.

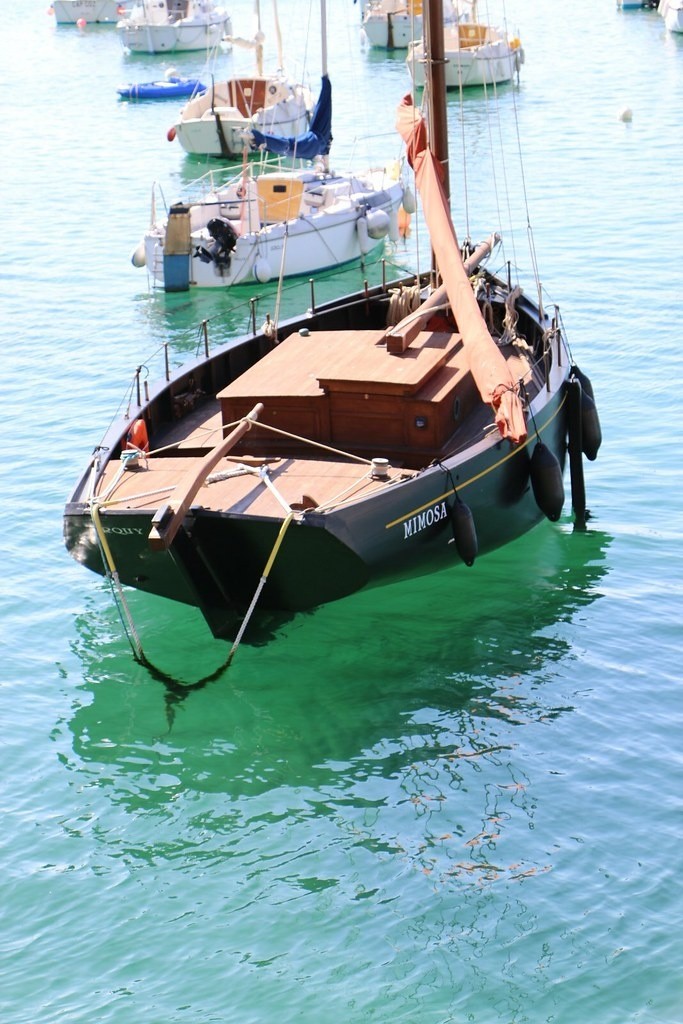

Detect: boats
left=48, top=1, right=683, bottom=694
